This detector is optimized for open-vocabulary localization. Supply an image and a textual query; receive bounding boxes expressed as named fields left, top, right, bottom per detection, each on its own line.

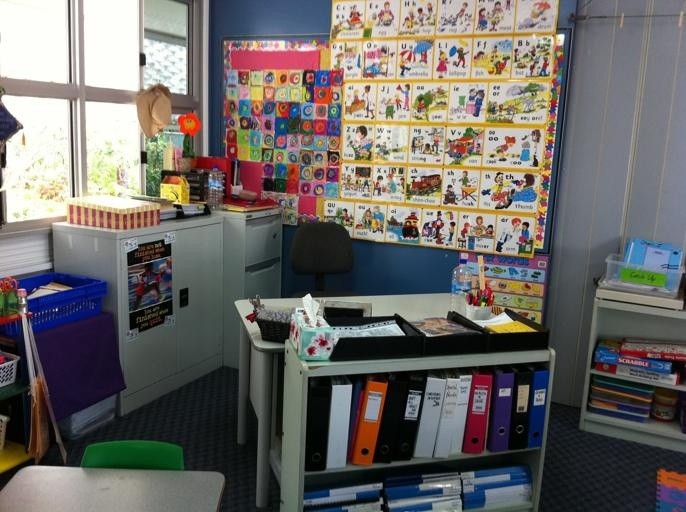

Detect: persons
left=159, top=257, right=172, bottom=296
left=135, top=261, right=162, bottom=309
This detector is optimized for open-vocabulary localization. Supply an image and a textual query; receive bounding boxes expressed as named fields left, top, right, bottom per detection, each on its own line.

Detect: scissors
left=1, top=277, right=17, bottom=293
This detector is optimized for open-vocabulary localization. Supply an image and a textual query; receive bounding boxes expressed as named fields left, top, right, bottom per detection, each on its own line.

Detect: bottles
left=451, top=257, right=474, bottom=316
left=161, top=142, right=175, bottom=171
left=208, top=165, right=224, bottom=213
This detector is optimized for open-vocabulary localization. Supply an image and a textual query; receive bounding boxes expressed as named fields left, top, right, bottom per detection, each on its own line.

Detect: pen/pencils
left=467, top=287, right=495, bottom=307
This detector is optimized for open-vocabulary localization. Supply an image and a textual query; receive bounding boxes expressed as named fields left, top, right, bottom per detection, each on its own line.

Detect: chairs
left=288, top=221, right=359, bottom=299
left=80, top=440, right=185, bottom=471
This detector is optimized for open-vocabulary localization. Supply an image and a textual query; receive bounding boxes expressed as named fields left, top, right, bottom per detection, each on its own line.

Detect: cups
left=466, top=303, right=492, bottom=321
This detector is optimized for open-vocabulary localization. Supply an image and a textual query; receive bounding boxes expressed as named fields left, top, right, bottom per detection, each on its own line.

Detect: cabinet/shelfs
left=579, top=298, right=686, bottom=453
left=51, top=216, right=223, bottom=418
left=213, top=209, right=283, bottom=371
left=0, top=311, right=36, bottom=477
left=279, top=339, right=557, bottom=512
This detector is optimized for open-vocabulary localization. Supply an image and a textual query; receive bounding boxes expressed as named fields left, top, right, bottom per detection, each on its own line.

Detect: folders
left=306, top=363, right=549, bottom=471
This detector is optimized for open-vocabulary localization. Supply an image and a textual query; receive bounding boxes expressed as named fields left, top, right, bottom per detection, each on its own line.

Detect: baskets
left=254, top=311, right=290, bottom=344
left=0, top=271, right=108, bottom=339
left=0, top=415, right=11, bottom=450
left=0, top=350, right=20, bottom=390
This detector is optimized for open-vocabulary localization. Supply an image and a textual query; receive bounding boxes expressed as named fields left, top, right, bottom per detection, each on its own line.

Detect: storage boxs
left=68, top=193, right=162, bottom=230
left=605, top=253, right=686, bottom=298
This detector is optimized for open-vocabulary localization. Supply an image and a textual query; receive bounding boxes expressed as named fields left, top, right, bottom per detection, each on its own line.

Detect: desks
left=0, top=465, right=225, bottom=512
left=234, top=293, right=467, bottom=508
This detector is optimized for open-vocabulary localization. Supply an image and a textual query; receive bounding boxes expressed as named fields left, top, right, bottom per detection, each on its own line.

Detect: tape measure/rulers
left=478, top=255, right=485, bottom=291
